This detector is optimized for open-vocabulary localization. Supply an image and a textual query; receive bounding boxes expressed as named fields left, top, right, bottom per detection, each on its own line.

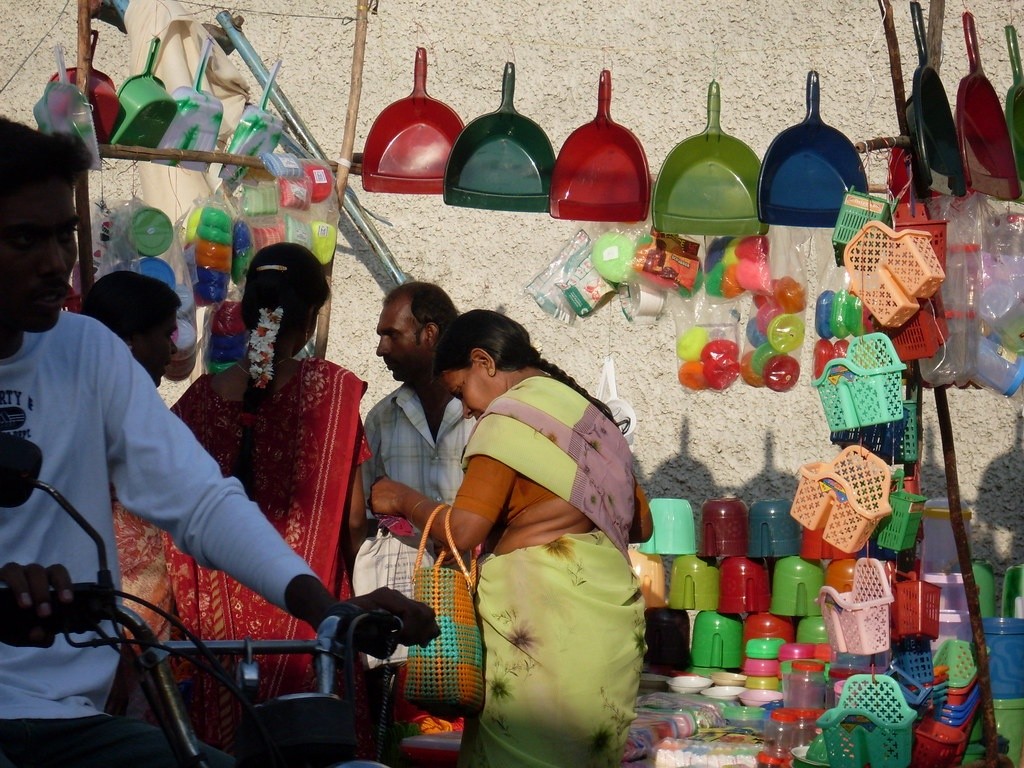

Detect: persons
left=368, top=308, right=654, bottom=768
left=363, top=281, right=479, bottom=569
left=0, top=118, right=442, bottom=768
left=156, top=241, right=373, bottom=760
left=79, top=270, right=181, bottom=725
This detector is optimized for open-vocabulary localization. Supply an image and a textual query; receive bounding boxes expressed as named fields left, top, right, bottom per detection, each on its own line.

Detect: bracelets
left=411, top=500, right=424, bottom=523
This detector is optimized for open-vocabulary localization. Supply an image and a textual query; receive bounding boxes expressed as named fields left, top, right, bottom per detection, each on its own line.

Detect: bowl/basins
left=639, top=672, right=783, bottom=707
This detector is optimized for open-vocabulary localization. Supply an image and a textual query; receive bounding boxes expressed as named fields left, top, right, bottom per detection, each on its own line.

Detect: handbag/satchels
left=404, top=503, right=485, bottom=722
left=352, top=517, right=437, bottom=669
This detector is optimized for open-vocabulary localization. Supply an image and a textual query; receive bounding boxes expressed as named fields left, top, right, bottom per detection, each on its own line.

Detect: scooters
left=0, top=431, right=441, bottom=768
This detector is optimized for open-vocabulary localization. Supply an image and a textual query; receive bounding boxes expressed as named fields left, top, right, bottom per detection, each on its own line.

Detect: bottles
left=757, top=661, right=828, bottom=768
left=919, top=215, right=1024, bottom=396
